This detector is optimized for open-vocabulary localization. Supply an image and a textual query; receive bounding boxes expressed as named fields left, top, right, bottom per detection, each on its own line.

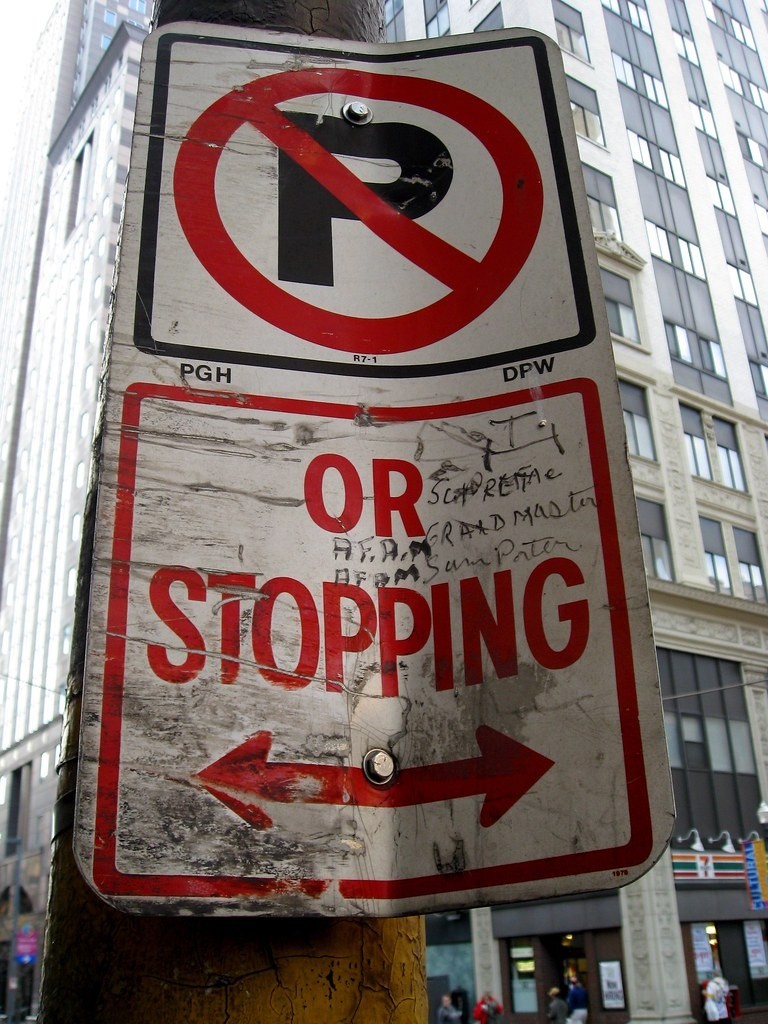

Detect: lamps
left=756, top=801, right=768, bottom=824
left=709, top=830, right=735, bottom=854
left=677, top=828, right=704, bottom=852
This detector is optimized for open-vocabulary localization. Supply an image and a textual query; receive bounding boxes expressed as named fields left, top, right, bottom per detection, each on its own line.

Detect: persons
left=472, top=991, right=503, bottom=1024
left=701, top=969, right=732, bottom=1024
left=566, top=977, right=588, bottom=1024
left=485, top=1002, right=508, bottom=1024
left=545, top=987, right=568, bottom=1024
left=435, top=992, right=462, bottom=1024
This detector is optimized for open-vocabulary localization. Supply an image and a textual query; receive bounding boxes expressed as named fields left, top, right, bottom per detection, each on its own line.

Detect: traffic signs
left=92, top=381, right=652, bottom=899
